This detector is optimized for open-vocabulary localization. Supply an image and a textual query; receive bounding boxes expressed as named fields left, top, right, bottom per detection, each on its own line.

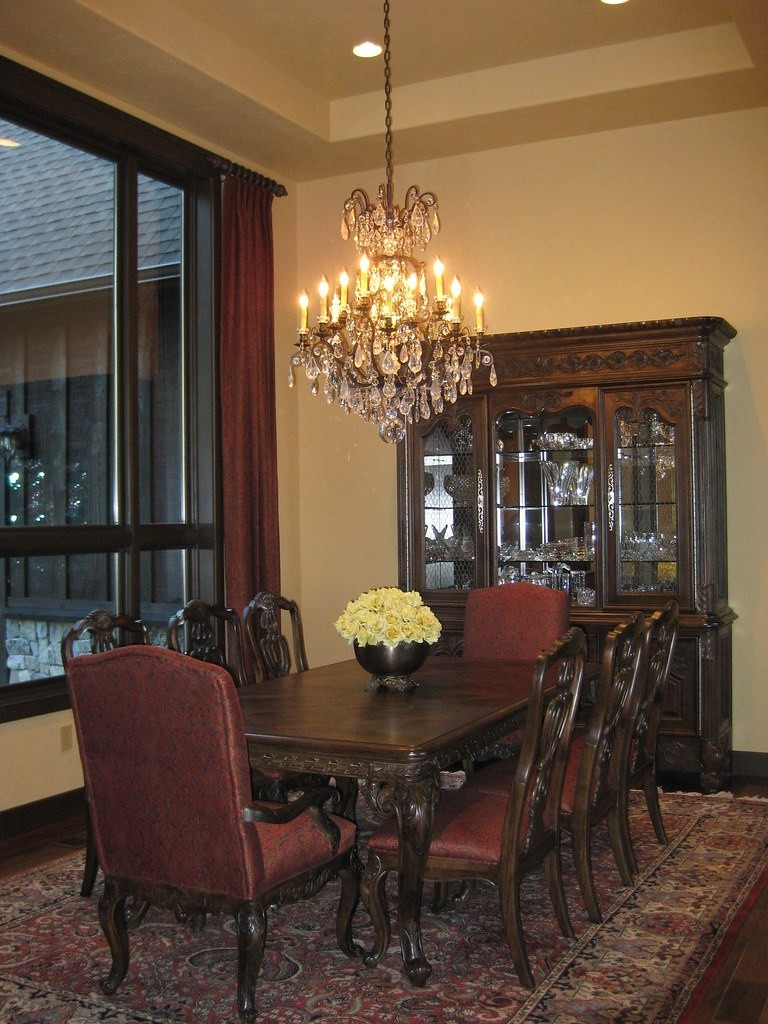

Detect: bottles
left=619, top=410, right=674, bottom=446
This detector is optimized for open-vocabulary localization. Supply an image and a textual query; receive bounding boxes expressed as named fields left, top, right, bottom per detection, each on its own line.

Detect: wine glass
left=622, top=530, right=677, bottom=561
left=624, top=450, right=675, bottom=479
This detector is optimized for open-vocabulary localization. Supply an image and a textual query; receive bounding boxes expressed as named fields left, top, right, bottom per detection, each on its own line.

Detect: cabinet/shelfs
left=397, top=315, right=740, bottom=793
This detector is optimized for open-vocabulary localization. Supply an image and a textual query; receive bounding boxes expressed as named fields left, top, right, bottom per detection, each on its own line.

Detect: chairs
left=432, top=582, right=571, bottom=659
left=243, top=591, right=359, bottom=823
left=356, top=602, right=582, bottom=991
left=646, top=600, right=680, bottom=845
left=65, top=644, right=366, bottom=1024
left=61, top=608, right=150, bottom=900
left=166, top=599, right=330, bottom=802
left=501, top=612, right=660, bottom=923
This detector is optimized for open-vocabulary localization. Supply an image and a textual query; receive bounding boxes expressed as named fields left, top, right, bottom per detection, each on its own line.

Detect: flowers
left=333, top=587, right=442, bottom=648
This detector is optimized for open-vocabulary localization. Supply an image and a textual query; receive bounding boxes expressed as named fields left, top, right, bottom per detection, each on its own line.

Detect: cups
left=540, top=460, right=593, bottom=506
left=499, top=521, right=597, bottom=561
left=622, top=578, right=677, bottom=592
left=498, top=563, right=596, bottom=606
left=536, top=432, right=594, bottom=450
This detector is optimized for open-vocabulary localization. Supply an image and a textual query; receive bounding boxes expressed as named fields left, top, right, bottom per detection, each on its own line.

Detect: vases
left=353, top=638, right=430, bottom=676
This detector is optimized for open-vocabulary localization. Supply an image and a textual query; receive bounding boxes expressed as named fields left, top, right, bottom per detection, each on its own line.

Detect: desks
left=236, top=658, right=603, bottom=987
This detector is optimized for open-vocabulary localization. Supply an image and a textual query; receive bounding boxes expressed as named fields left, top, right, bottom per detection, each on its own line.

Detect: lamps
left=283, top=0, right=497, bottom=446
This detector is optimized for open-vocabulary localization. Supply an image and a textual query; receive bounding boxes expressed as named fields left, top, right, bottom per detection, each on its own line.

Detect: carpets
left=0, top=768, right=768, bottom=1024
